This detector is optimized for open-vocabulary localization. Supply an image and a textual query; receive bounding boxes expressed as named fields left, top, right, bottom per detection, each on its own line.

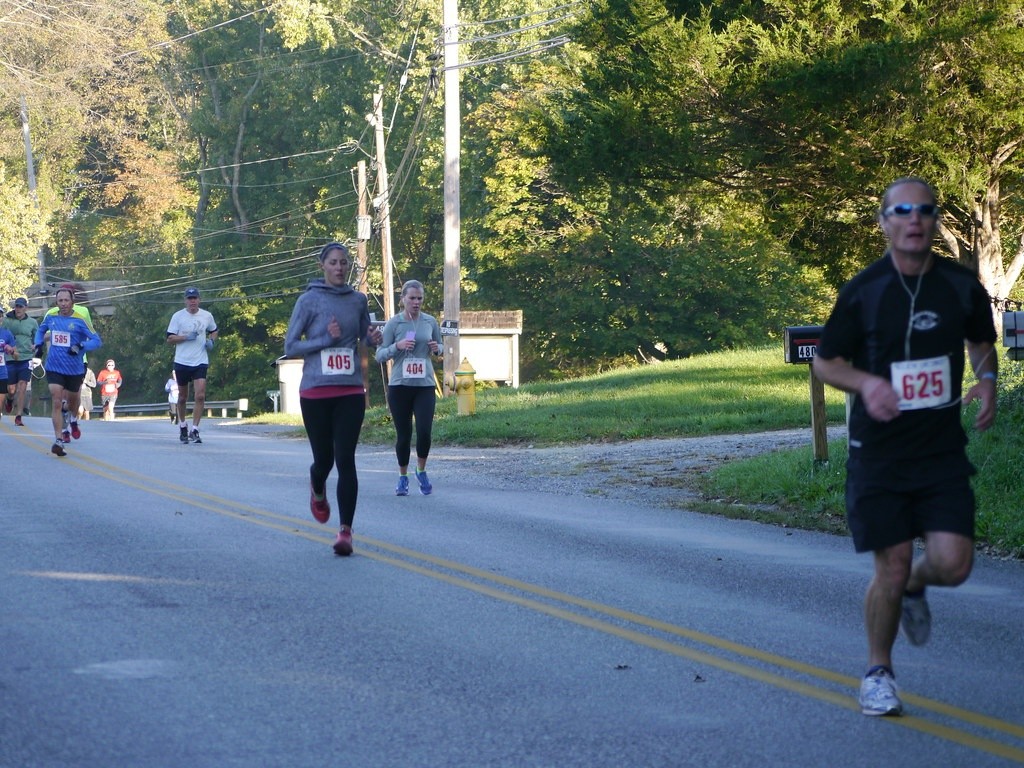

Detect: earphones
left=881, top=221, right=886, bottom=233
left=402, top=297, right=405, bottom=304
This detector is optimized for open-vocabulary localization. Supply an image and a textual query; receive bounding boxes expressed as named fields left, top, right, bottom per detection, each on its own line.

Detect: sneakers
left=311, top=483, right=330, bottom=523
left=15, top=415, right=24, bottom=426
left=61, top=400, right=69, bottom=428
left=52, top=439, right=67, bottom=456
left=395, top=472, right=410, bottom=495
left=70, top=420, right=80, bottom=439
left=6, top=397, right=14, bottom=413
left=902, top=588, right=931, bottom=646
left=860, top=665, right=902, bottom=717
left=189, top=429, right=201, bottom=443
left=333, top=527, right=353, bottom=555
left=62, top=431, right=71, bottom=443
left=180, top=423, right=189, bottom=443
left=415, top=466, right=432, bottom=495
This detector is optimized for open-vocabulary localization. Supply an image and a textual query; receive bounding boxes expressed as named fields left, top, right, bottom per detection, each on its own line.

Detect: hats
left=186, top=287, right=199, bottom=298
left=15, top=297, right=27, bottom=306
left=61, top=284, right=76, bottom=292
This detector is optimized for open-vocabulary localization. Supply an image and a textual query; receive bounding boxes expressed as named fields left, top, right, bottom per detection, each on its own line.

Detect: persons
left=77, top=368, right=96, bottom=421
left=167, top=288, right=218, bottom=444
left=376, top=280, right=443, bottom=498
left=2, top=298, right=39, bottom=426
left=97, top=359, right=122, bottom=423
left=165, top=370, right=179, bottom=425
left=285, top=243, right=383, bottom=556
left=0, top=308, right=18, bottom=418
left=43, top=284, right=98, bottom=443
left=812, top=177, right=998, bottom=717
left=33, top=288, right=102, bottom=456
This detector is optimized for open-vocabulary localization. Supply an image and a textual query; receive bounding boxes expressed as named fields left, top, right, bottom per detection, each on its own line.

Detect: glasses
left=884, top=202, right=939, bottom=217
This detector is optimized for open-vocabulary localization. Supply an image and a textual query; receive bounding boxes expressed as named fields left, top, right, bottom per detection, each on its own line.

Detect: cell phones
left=406, top=331, right=416, bottom=340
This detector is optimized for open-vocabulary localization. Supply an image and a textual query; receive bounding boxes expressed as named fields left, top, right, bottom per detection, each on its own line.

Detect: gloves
left=187, top=331, right=199, bottom=340
left=205, top=339, right=214, bottom=351
left=68, top=343, right=84, bottom=355
left=35, top=345, right=44, bottom=358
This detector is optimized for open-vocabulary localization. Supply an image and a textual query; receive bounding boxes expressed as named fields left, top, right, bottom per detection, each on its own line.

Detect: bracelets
left=981, top=373, right=997, bottom=384
left=11, top=349, right=14, bottom=355
